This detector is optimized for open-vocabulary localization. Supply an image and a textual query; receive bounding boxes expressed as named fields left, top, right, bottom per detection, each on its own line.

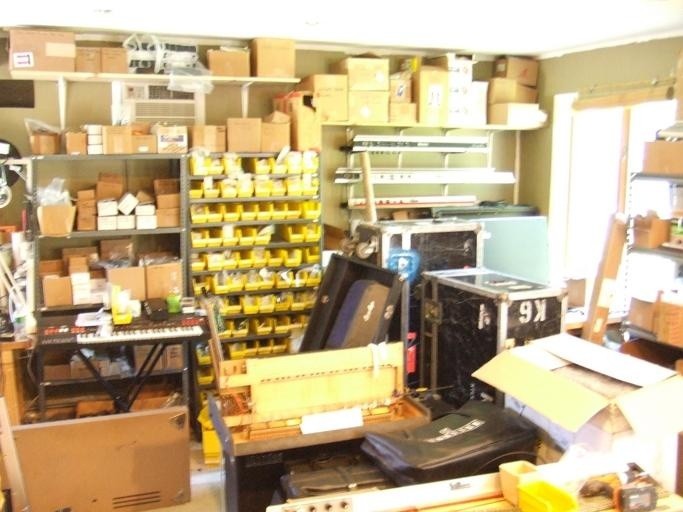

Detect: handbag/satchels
left=362, top=400, right=540, bottom=486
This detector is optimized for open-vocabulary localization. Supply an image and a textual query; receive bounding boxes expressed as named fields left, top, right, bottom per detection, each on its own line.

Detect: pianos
left=33, top=314, right=212, bottom=351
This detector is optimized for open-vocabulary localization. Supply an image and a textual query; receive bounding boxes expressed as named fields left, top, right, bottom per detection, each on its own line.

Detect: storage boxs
left=633, top=214, right=670, bottom=248
left=413, top=65, right=448, bottom=124
left=43, top=364, right=70, bottom=380
left=643, top=142, right=683, bottom=174
left=390, top=103, right=417, bottom=122
left=97, top=198, right=118, bottom=216
left=471, top=332, right=683, bottom=453
left=37, top=205, right=77, bottom=238
left=97, top=216, right=116, bottom=230
left=163, top=345, right=183, bottom=369
left=297, top=74, right=348, bottom=121
left=117, top=216, right=135, bottom=230
left=102, top=122, right=157, bottom=154
left=390, top=79, right=413, bottom=102
left=208, top=50, right=250, bottom=77
left=65, top=130, right=86, bottom=153
left=39, top=260, right=73, bottom=304
left=488, top=103, right=538, bottom=124
left=153, top=178, right=180, bottom=209
left=137, top=188, right=154, bottom=204
left=135, top=205, right=155, bottom=215
left=248, top=39, right=295, bottom=77
left=152, top=123, right=188, bottom=153
left=76, top=47, right=101, bottom=72
left=493, top=54, right=538, bottom=87
left=273, top=97, right=320, bottom=150
left=262, top=123, right=290, bottom=152
left=489, top=77, right=539, bottom=104
left=136, top=215, right=156, bottom=230
left=144, top=251, right=183, bottom=299
left=653, top=289, right=683, bottom=348
left=428, top=52, right=474, bottom=124
left=107, top=266, right=146, bottom=301
left=348, top=91, right=389, bottom=122
left=329, top=51, right=390, bottom=91
left=227, top=118, right=262, bottom=153
left=71, top=358, right=109, bottom=379
left=29, top=133, right=61, bottom=154
left=8, top=30, right=75, bottom=72
left=118, top=191, right=139, bottom=215
left=188, top=125, right=226, bottom=152
left=156, top=208, right=180, bottom=227
left=77, top=189, right=97, bottom=216
left=101, top=48, right=128, bottom=73
left=627, top=297, right=656, bottom=333
left=134, top=346, right=162, bottom=371
left=96, top=172, right=124, bottom=198
left=263, top=110, right=290, bottom=123
left=77, top=216, right=96, bottom=230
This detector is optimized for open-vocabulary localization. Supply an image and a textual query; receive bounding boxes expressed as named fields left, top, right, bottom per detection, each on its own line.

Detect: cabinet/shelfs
left=32, top=150, right=324, bottom=444
